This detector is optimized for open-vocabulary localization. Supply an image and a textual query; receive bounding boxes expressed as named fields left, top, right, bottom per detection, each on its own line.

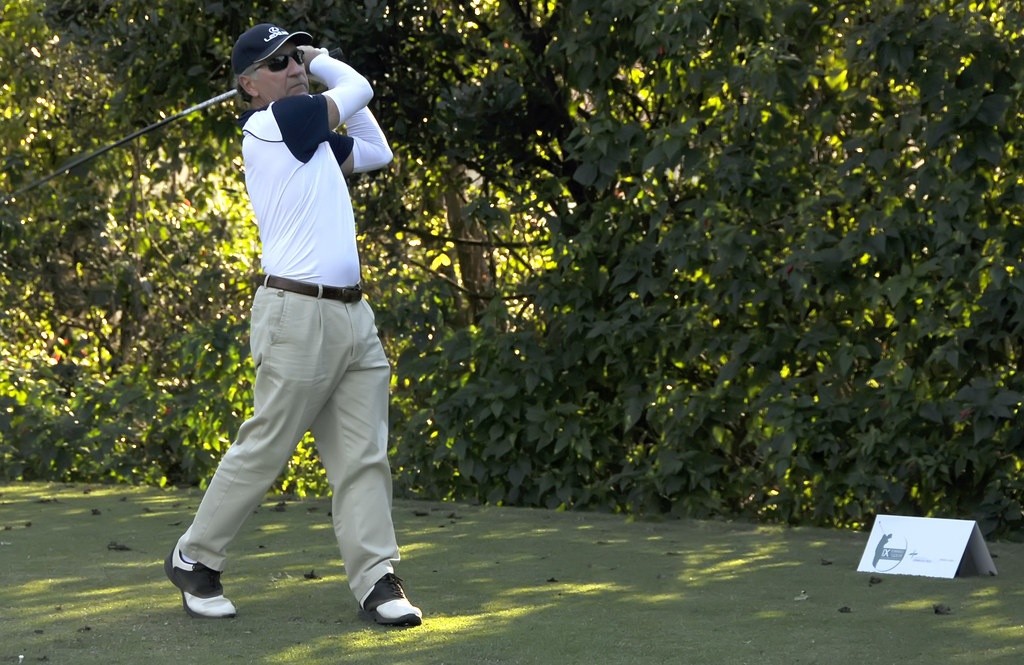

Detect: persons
left=164, top=24, right=422, bottom=628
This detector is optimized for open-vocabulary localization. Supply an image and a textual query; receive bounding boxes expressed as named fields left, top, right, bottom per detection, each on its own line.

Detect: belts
left=256, top=273, right=362, bottom=303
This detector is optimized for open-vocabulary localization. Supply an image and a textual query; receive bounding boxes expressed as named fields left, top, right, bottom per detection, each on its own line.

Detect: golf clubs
left=0, top=46, right=346, bottom=205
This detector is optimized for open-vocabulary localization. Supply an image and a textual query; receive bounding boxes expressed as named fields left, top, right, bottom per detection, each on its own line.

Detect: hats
left=231, top=24, right=313, bottom=75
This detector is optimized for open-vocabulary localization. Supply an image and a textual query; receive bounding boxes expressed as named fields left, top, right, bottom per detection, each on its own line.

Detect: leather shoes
left=164, top=537, right=236, bottom=619
left=358, top=574, right=423, bottom=625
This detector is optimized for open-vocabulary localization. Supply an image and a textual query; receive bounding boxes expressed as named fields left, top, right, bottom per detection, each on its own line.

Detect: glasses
left=254, top=50, right=304, bottom=72
